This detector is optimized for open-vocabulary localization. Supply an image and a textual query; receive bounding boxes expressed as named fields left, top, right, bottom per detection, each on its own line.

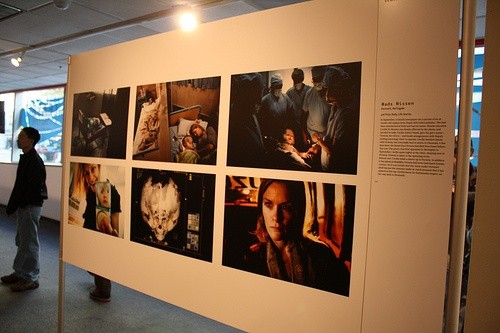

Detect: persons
left=235, top=178, right=350, bottom=297
left=69, top=162, right=122, bottom=238
left=0, top=126, right=49, bottom=293
left=130, top=62, right=360, bottom=177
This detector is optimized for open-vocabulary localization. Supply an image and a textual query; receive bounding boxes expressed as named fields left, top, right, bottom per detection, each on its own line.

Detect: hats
left=311, top=65, right=329, bottom=82
left=270, top=73, right=284, bottom=87
left=452, top=134, right=475, bottom=153
left=291, top=70, right=305, bottom=80
left=238, top=72, right=264, bottom=99
left=320, top=67, right=353, bottom=92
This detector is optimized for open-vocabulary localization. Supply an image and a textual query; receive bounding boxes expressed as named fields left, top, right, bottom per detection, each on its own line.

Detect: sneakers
left=89, top=286, right=111, bottom=303
left=10, top=278, right=40, bottom=292
left=2, top=272, right=26, bottom=284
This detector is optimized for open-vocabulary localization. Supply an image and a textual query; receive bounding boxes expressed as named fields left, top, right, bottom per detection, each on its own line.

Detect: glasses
left=313, top=78, right=324, bottom=83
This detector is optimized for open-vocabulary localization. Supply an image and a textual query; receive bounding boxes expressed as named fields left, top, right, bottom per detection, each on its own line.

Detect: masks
left=293, top=82, right=302, bottom=91
left=323, top=92, right=334, bottom=107
left=312, top=82, right=323, bottom=90
left=273, top=88, right=284, bottom=97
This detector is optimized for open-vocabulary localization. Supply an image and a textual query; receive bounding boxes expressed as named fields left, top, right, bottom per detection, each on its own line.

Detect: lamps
left=10, top=50, right=26, bottom=67
